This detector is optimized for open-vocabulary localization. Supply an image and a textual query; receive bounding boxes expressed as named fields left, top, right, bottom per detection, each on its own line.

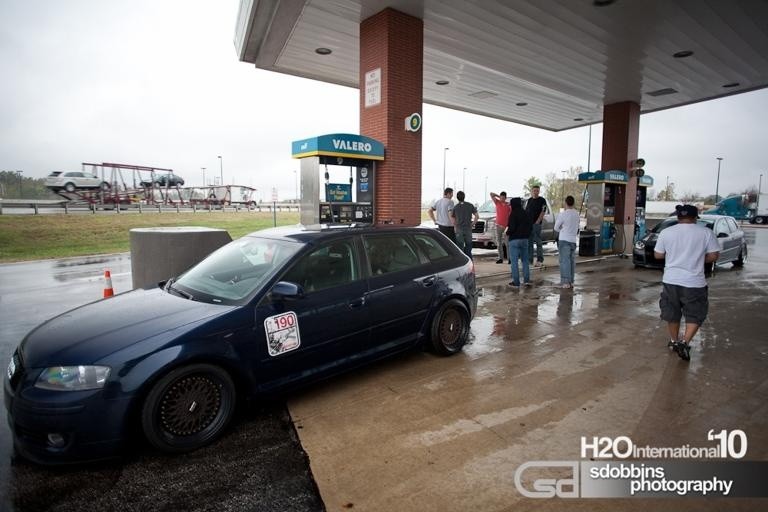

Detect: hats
left=678, top=204, right=701, bottom=219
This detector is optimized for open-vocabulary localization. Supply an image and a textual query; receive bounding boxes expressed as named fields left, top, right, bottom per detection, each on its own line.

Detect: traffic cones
left=103, top=271, right=114, bottom=297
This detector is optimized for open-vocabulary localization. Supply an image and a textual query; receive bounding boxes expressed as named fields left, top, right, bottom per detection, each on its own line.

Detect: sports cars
left=4, top=225, right=484, bottom=452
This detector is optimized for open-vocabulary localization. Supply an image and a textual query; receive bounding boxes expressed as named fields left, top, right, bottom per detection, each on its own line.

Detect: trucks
left=703, top=194, right=768, bottom=224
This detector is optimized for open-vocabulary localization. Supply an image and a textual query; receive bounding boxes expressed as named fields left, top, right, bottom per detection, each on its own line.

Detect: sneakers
left=668, top=339, right=677, bottom=350
left=524, top=280, right=534, bottom=286
left=496, top=259, right=503, bottom=263
left=675, top=339, right=691, bottom=361
left=552, top=283, right=570, bottom=288
left=570, top=283, right=574, bottom=287
left=508, top=281, right=520, bottom=288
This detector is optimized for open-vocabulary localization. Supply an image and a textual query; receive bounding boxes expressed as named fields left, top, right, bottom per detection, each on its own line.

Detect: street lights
left=463, top=168, right=467, bottom=191
left=667, top=176, right=669, bottom=190
left=442, top=147, right=449, bottom=195
left=218, top=155, right=223, bottom=185
left=759, top=173, right=763, bottom=194
left=716, top=157, right=723, bottom=204
left=201, top=168, right=206, bottom=186
left=561, top=170, right=566, bottom=209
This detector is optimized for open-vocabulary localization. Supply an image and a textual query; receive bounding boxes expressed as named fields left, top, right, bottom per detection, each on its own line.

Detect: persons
left=428, top=188, right=455, bottom=238
left=555, top=195, right=580, bottom=288
left=453, top=191, right=478, bottom=258
left=506, top=197, right=532, bottom=286
left=527, top=187, right=547, bottom=266
left=654, top=205, right=720, bottom=363
left=491, top=191, right=511, bottom=264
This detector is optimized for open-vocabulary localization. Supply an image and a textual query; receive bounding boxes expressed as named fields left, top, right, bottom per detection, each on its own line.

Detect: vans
left=467, top=197, right=559, bottom=249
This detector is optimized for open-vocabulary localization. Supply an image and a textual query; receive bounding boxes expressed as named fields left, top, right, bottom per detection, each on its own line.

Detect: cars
left=633, top=214, right=747, bottom=273
left=140, top=173, right=184, bottom=188
left=44, top=170, right=111, bottom=192
